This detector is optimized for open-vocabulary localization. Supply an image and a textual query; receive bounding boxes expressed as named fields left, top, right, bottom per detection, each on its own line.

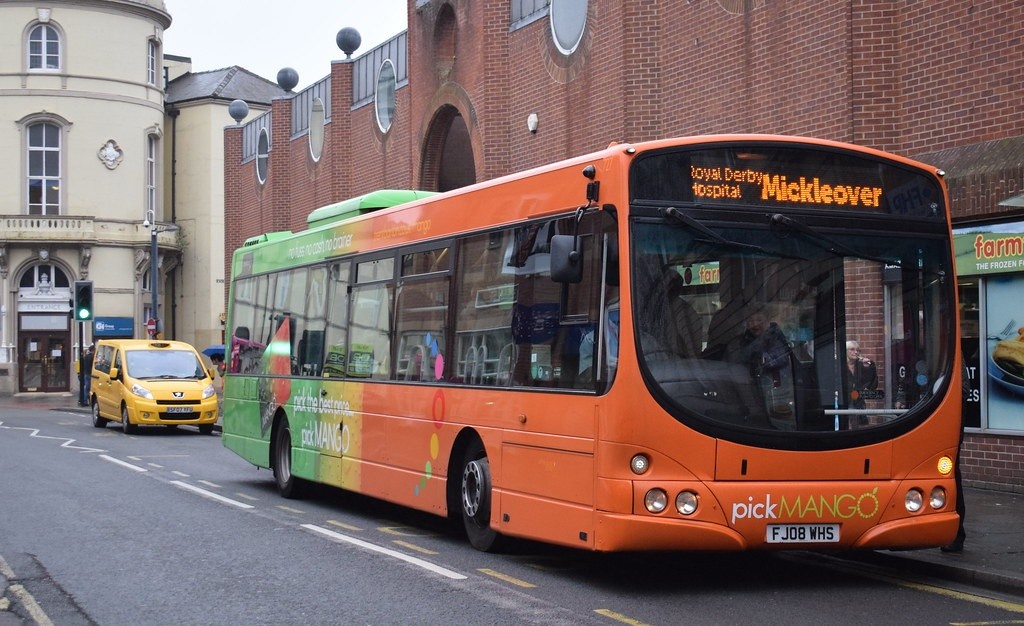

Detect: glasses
left=848, top=348, right=861, bottom=353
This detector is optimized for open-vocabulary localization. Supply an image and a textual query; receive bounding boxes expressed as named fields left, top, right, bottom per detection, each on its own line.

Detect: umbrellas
left=201, top=344, right=225, bottom=357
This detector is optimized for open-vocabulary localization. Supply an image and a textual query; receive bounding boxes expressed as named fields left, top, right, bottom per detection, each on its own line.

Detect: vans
left=89, top=340, right=218, bottom=435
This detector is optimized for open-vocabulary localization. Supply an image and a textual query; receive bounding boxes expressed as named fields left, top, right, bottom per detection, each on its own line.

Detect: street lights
left=151, top=225, right=178, bottom=340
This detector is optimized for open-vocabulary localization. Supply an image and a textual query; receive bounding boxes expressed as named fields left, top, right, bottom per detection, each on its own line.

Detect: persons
left=704, top=282, right=768, bottom=361
left=719, top=298, right=798, bottom=434
left=845, top=340, right=878, bottom=432
left=209, top=352, right=227, bottom=416
left=75, top=344, right=96, bottom=407
left=352, top=247, right=706, bottom=391
left=894, top=309, right=971, bottom=552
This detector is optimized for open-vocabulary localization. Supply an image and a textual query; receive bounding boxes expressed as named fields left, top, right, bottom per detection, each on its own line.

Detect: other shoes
left=84, top=401, right=89, bottom=406
left=940, top=545, right=963, bottom=552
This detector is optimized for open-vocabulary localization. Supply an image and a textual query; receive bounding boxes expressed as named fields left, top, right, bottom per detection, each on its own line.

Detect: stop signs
left=147, top=318, right=156, bottom=335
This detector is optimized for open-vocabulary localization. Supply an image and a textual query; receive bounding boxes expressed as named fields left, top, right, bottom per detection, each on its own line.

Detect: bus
left=221, top=134, right=963, bottom=554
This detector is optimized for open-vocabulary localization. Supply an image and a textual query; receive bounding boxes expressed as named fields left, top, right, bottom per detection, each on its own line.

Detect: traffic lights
left=74, top=281, right=93, bottom=321
